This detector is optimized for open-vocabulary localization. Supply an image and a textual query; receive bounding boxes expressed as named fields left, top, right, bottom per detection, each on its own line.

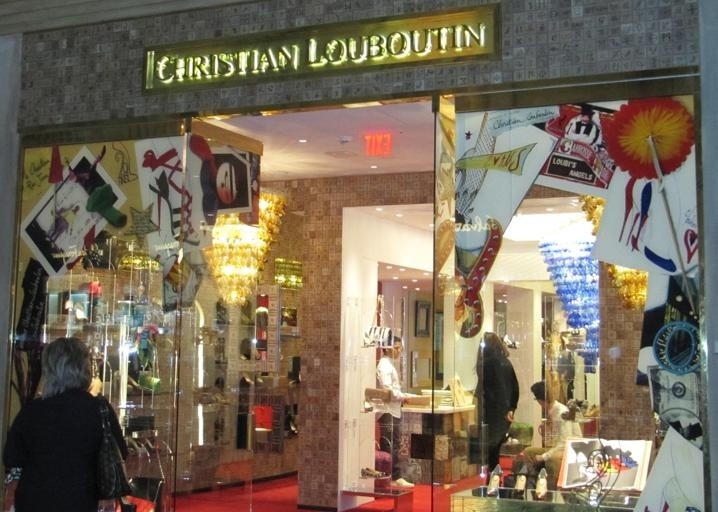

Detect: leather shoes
left=361, top=467, right=386, bottom=478
left=514, top=463, right=528, bottom=489
left=487, top=464, right=503, bottom=497
left=535, top=467, right=548, bottom=499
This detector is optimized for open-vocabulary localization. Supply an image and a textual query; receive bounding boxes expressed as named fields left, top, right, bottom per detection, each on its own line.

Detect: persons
left=566, top=103, right=600, bottom=147
left=0, top=337, right=131, bottom=510
left=471, top=331, right=521, bottom=487
left=375, top=335, right=417, bottom=487
left=524, top=381, right=585, bottom=477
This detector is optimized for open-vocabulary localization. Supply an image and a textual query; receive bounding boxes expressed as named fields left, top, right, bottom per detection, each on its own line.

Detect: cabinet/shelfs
left=36, top=263, right=305, bottom=495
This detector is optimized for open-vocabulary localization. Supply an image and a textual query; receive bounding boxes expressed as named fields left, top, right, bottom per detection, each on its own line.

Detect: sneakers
left=389, top=477, right=415, bottom=488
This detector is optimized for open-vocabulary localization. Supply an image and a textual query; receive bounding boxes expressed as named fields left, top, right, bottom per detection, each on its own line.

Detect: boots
left=561, top=399, right=589, bottom=420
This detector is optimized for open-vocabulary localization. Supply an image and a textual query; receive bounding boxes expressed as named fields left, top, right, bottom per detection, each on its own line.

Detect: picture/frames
left=416, top=300, right=431, bottom=337
left=411, top=349, right=433, bottom=388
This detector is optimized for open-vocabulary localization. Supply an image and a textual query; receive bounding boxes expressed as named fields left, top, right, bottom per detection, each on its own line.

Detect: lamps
left=196, top=184, right=288, bottom=306
left=535, top=194, right=650, bottom=375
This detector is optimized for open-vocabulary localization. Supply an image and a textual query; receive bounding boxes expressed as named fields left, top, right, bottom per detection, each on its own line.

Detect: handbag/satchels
left=562, top=328, right=586, bottom=350
left=97, top=396, right=131, bottom=501
left=364, top=388, right=393, bottom=402
left=363, top=326, right=392, bottom=346
